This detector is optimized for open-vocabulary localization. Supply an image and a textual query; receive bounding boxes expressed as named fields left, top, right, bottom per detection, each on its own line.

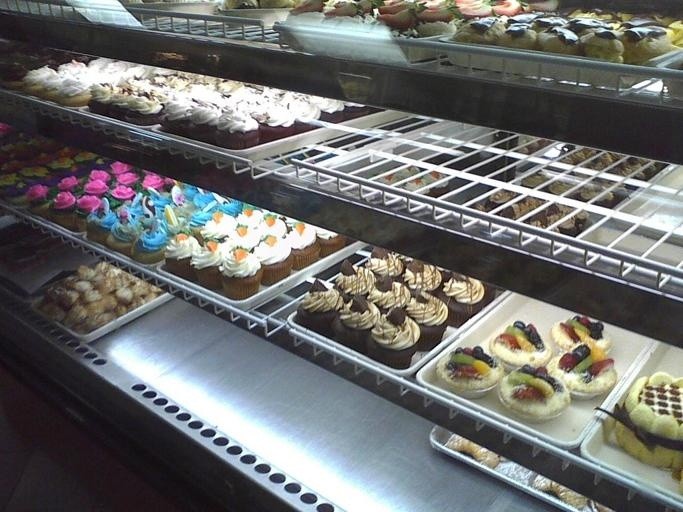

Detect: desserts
left=289, top=0, right=683, bottom=62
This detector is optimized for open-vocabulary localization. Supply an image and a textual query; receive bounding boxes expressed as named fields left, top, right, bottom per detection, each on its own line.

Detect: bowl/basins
left=440, top=312, right=620, bottom=424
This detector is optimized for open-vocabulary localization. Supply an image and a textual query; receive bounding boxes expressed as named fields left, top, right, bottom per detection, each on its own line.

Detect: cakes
left=593, top=372, right=683, bottom=470
left=376, top=164, right=452, bottom=198
left=0, top=120, right=175, bottom=232
left=487, top=319, right=553, bottom=369
left=464, top=146, right=668, bottom=239
left=163, top=207, right=345, bottom=300
left=157, top=77, right=386, bottom=150
left=86, top=184, right=243, bottom=264
left=435, top=346, right=505, bottom=400
left=296, top=246, right=495, bottom=370
left=497, top=363, right=571, bottom=424
left=22, top=55, right=246, bottom=126
left=551, top=313, right=612, bottom=352
left=545, top=337, right=618, bottom=400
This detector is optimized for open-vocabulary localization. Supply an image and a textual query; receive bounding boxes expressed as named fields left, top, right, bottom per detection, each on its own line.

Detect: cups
left=298, top=254, right=489, bottom=370
left=161, top=197, right=345, bottom=299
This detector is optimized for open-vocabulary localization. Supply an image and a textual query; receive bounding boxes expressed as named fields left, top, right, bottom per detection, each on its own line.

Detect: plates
left=218, top=6, right=290, bottom=27
